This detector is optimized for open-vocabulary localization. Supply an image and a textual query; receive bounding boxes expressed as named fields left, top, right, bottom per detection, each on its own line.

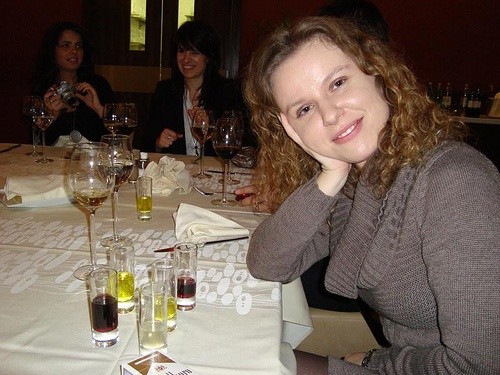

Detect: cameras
left=52, top=81, right=82, bottom=107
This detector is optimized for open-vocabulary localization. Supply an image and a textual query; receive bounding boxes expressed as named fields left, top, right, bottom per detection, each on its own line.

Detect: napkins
left=142, top=154, right=195, bottom=196
left=0, top=173, right=118, bottom=208
left=172, top=204, right=250, bottom=245
left=56, top=135, right=94, bottom=151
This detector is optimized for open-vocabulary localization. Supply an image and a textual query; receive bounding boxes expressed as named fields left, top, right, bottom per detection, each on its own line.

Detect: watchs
left=362, top=349, right=376, bottom=367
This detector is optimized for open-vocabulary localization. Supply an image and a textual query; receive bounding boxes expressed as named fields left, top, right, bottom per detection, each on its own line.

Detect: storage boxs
left=119, top=351, right=193, bottom=375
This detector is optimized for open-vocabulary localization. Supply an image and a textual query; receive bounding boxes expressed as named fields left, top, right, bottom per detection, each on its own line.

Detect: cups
left=138, top=281, right=168, bottom=358
left=137, top=177, right=152, bottom=222
left=152, top=259, right=177, bottom=332
left=88, top=268, right=121, bottom=347
left=174, top=243, right=198, bottom=310
left=107, top=242, right=135, bottom=315
left=103, top=102, right=138, bottom=133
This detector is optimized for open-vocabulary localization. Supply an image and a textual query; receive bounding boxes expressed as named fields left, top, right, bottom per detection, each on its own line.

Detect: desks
left=0, top=142, right=314, bottom=375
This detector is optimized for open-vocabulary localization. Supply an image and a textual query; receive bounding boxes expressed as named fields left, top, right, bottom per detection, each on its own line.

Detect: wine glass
left=96, top=134, right=134, bottom=247
left=191, top=109, right=244, bottom=207
left=67, top=141, right=115, bottom=281
left=22, top=95, right=43, bottom=157
left=33, top=100, right=55, bottom=164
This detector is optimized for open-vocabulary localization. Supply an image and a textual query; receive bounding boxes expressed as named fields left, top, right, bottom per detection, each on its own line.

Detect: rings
left=168, top=135, right=172, bottom=139
left=50, top=100, right=53, bottom=102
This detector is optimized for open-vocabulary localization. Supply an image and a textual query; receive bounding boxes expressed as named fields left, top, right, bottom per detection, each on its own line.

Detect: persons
left=18, top=22, right=113, bottom=146
left=242, top=16, right=500, bottom=375
left=132, top=21, right=249, bottom=156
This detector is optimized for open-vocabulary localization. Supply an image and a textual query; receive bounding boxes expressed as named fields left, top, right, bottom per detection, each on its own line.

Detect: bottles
left=129, top=148, right=150, bottom=183
left=428, top=82, right=495, bottom=118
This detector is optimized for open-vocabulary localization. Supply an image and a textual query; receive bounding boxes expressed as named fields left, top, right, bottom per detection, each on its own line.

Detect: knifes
left=153, top=236, right=250, bottom=253
left=203, top=169, right=254, bottom=175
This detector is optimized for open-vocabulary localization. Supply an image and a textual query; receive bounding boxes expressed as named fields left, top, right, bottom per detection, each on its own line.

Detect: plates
left=181, top=224, right=257, bottom=269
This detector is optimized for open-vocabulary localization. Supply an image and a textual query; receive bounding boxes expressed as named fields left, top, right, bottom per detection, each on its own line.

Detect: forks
left=194, top=185, right=237, bottom=196
